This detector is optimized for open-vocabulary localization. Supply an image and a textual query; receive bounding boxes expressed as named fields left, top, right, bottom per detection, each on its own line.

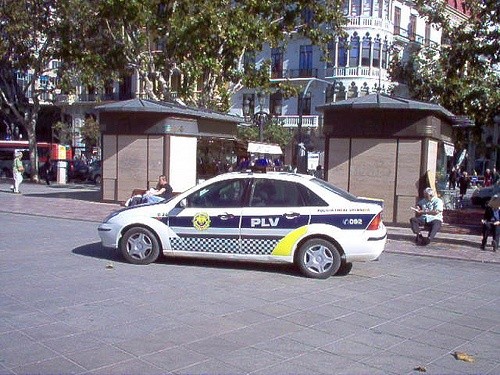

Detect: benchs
left=419, top=209, right=493, bottom=237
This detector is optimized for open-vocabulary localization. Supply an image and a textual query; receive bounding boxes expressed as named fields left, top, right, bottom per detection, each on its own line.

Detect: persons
left=492, top=168, right=500, bottom=184
left=480, top=195, right=500, bottom=251
left=455, top=165, right=462, bottom=186
left=473, top=170, right=477, bottom=176
left=411, top=188, right=443, bottom=245
left=449, top=167, right=456, bottom=190
left=459, top=172, right=470, bottom=199
left=10, top=152, right=24, bottom=194
left=314, top=166, right=323, bottom=179
left=485, top=169, right=493, bottom=187
left=44, top=156, right=52, bottom=185
left=81, top=154, right=96, bottom=165
left=142, top=175, right=172, bottom=204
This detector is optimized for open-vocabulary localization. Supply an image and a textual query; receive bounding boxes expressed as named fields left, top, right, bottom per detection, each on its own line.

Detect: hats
left=15, top=152, right=22, bottom=157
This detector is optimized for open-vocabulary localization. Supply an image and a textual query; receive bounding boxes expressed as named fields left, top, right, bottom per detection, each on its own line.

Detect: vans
left=0, top=149, right=31, bottom=177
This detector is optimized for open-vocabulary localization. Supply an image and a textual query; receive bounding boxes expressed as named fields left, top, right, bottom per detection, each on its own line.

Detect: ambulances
left=98, top=159, right=387, bottom=280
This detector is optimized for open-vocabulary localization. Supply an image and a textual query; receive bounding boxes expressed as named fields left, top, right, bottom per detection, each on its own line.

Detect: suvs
left=38, top=159, right=89, bottom=183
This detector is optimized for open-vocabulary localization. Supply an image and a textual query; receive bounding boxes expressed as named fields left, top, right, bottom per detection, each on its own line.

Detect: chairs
left=253, top=182, right=276, bottom=207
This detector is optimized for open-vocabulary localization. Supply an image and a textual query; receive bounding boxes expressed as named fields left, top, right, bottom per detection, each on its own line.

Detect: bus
left=0, top=140, right=72, bottom=181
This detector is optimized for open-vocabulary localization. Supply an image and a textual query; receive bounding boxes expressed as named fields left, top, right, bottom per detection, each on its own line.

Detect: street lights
left=247, top=89, right=271, bottom=159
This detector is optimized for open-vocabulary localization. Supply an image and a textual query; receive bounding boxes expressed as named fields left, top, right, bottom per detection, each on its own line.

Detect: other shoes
left=480, top=244, right=485, bottom=250
left=423, top=237, right=430, bottom=245
left=492, top=240, right=497, bottom=251
left=416, top=235, right=423, bottom=245
left=10, top=188, right=14, bottom=190
left=13, top=192, right=20, bottom=194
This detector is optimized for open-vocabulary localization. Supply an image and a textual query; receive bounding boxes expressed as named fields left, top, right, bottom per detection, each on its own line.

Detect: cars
left=470, top=179, right=500, bottom=208
left=86, top=160, right=103, bottom=184
